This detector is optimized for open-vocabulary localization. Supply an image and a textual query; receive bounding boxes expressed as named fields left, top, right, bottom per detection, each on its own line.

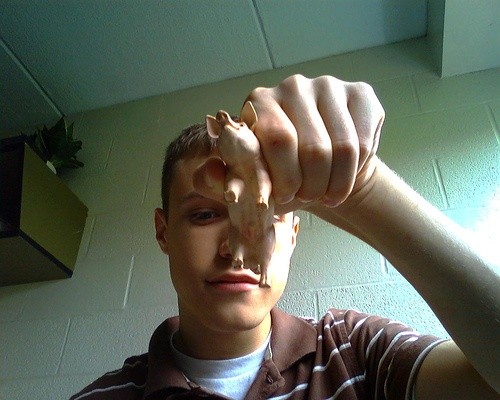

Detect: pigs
left=206, top=101, right=275, bottom=288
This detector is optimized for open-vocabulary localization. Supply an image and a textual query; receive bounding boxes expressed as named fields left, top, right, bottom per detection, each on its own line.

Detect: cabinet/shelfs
left=1, top=136, right=89, bottom=288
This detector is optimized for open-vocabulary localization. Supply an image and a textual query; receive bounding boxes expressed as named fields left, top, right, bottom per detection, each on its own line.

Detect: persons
left=64, top=69, right=500, bottom=400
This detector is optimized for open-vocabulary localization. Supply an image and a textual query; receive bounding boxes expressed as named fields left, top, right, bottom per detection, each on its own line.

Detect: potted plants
left=24, top=115, right=86, bottom=176
